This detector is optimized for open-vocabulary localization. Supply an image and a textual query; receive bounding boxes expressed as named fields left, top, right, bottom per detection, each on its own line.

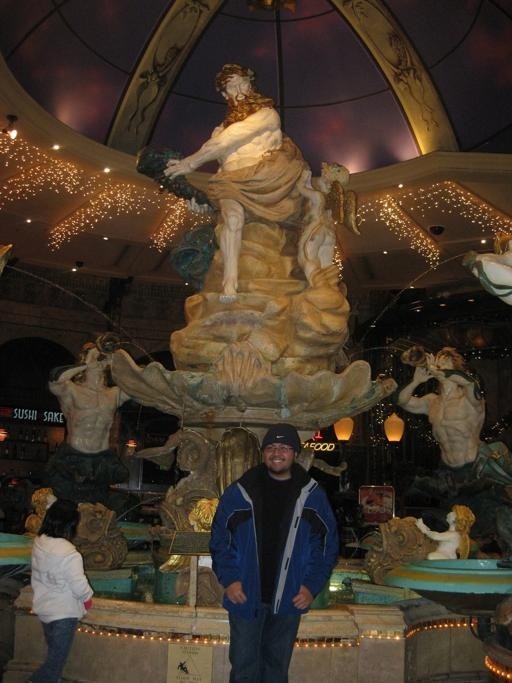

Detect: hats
left=259, top=423, right=302, bottom=452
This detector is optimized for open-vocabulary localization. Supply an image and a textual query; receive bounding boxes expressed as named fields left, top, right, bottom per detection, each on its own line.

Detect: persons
left=398, top=346, right=512, bottom=521
left=209, top=424, right=340, bottom=683
left=43, top=342, right=129, bottom=485
left=23, top=498, right=94, bottom=683
left=163, top=62, right=311, bottom=302
left=296, top=161, right=350, bottom=287
left=415, top=504, right=476, bottom=561
